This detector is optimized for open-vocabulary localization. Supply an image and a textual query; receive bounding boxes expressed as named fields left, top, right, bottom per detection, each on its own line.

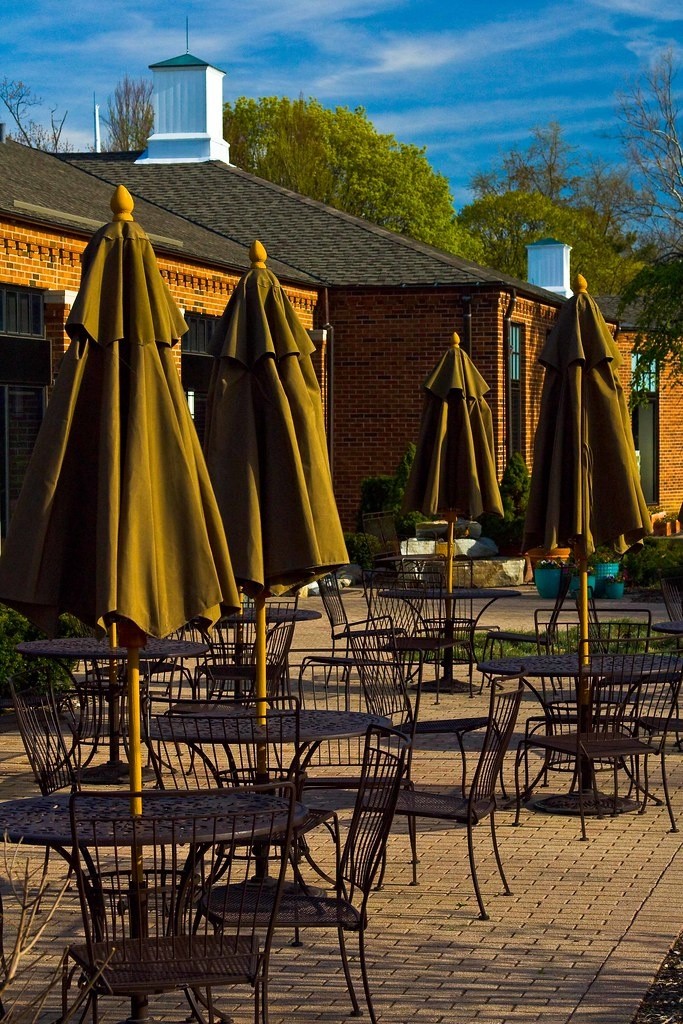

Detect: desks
left=179, top=608, right=322, bottom=710
left=0, top=790, right=310, bottom=1024
left=15, top=636, right=210, bottom=791
left=375, top=589, right=522, bottom=693
left=477, top=655, right=683, bottom=808
left=140, top=708, right=393, bottom=916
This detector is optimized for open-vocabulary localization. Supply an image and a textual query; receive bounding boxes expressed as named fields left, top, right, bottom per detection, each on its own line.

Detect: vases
left=569, top=577, right=596, bottom=598
left=606, top=582, right=625, bottom=599
left=591, top=562, right=619, bottom=597
left=535, top=568, right=571, bottom=599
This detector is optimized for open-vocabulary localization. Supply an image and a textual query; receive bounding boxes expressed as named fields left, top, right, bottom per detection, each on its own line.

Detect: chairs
left=9, top=513, right=683, bottom=1024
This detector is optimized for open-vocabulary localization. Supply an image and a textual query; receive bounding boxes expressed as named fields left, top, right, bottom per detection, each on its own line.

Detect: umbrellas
left=0, top=184, right=244, bottom=1024
left=400, top=331, right=505, bottom=685
left=516, top=274, right=653, bottom=795
left=198, top=240, right=349, bottom=883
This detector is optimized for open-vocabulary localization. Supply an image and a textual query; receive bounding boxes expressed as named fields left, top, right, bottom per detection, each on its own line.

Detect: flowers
left=604, top=570, right=628, bottom=585
left=536, top=558, right=567, bottom=568
left=571, top=563, right=597, bottom=576
left=586, top=546, right=621, bottom=565
